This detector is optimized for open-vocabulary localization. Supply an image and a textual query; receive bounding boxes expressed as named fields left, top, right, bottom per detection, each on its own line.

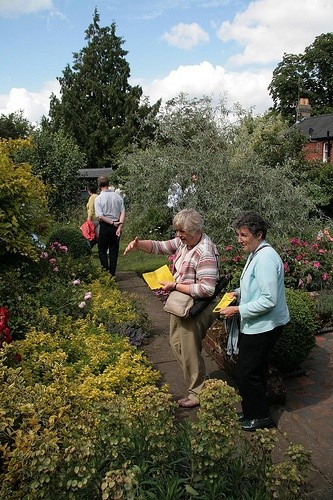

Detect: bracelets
left=113, top=221, right=114, bottom=225
left=174, top=283, right=178, bottom=290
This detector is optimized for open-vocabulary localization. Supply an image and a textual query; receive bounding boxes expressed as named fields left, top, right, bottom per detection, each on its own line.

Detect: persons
left=86, top=183, right=110, bottom=258
left=220, top=211, right=291, bottom=432
left=122, top=208, right=219, bottom=408
left=165, top=171, right=199, bottom=208
left=95, top=175, right=126, bottom=281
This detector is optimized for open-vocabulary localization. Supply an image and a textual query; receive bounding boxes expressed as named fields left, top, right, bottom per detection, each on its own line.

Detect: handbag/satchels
left=163, top=290, right=195, bottom=320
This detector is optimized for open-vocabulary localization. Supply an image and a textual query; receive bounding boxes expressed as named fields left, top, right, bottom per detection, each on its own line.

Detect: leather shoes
left=242, top=416, right=274, bottom=431
left=239, top=415, right=249, bottom=421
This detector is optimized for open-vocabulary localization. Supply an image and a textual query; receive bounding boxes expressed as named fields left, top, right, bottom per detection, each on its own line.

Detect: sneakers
left=178, top=398, right=199, bottom=407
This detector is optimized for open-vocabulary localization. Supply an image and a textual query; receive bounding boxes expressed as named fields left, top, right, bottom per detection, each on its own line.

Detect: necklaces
left=187, top=235, right=202, bottom=250
